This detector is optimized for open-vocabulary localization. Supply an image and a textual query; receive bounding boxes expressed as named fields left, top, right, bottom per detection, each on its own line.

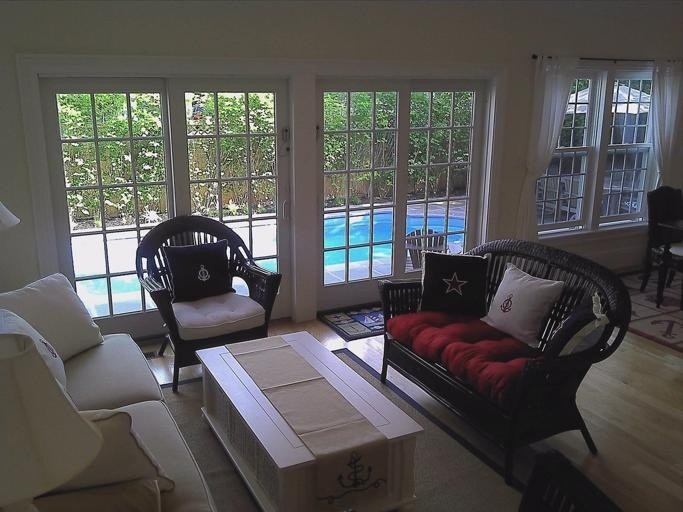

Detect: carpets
left=616, top=269, right=682, bottom=353
left=158, top=347, right=527, bottom=512
left=317, top=301, right=385, bottom=342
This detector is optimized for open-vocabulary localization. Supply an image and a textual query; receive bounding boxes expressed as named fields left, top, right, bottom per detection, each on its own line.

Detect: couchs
left=0, top=331, right=216, bottom=512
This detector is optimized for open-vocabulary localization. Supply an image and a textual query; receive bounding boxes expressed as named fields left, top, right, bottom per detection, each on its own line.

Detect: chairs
left=406, top=228, right=445, bottom=270
left=639, top=185, right=683, bottom=310
left=136, top=215, right=282, bottom=394
left=518, top=450, right=621, bottom=512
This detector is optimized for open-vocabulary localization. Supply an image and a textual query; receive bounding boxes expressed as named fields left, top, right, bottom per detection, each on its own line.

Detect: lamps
left=1, top=333, right=104, bottom=512
left=0, top=200, right=21, bottom=231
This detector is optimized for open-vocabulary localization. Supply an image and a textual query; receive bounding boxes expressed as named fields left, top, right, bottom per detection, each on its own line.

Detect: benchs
left=376, top=238, right=632, bottom=486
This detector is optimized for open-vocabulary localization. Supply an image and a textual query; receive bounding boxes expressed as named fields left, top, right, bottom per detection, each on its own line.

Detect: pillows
left=417, top=250, right=492, bottom=316
left=542, top=293, right=610, bottom=357
left=0, top=309, right=67, bottom=390
left=162, top=238, right=236, bottom=304
left=29, top=410, right=177, bottom=494
left=0, top=272, right=104, bottom=362
left=480, top=263, right=565, bottom=349
